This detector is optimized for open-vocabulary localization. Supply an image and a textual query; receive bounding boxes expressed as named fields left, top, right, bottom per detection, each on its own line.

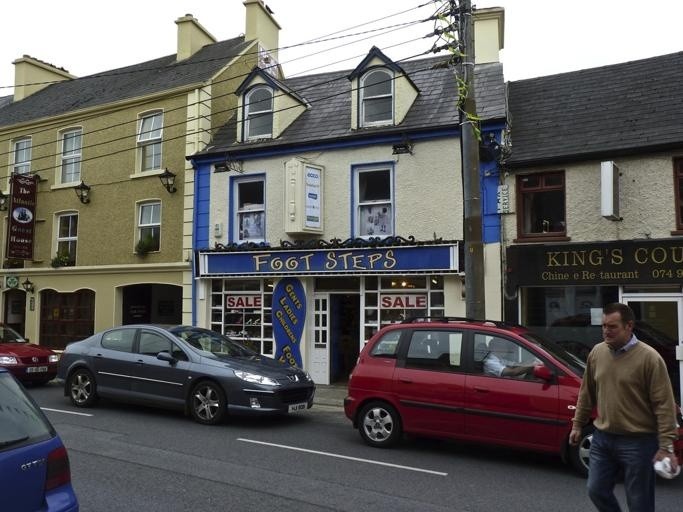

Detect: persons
left=568, top=302, right=680, bottom=512
left=483, top=339, right=538, bottom=379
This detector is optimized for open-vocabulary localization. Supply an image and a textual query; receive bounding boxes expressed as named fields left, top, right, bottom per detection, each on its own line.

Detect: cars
left=342, top=316, right=682, bottom=484
left=54, top=323, right=315, bottom=426
left=546, top=308, right=678, bottom=389
left=0, top=366, right=78, bottom=511
left=0, top=321, right=58, bottom=387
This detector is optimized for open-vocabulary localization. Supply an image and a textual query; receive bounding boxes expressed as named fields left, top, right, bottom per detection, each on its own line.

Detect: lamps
left=0, top=190, right=9, bottom=212
left=213, top=163, right=229, bottom=173
left=22, top=278, right=34, bottom=294
left=74, top=180, right=90, bottom=204
left=393, top=143, right=412, bottom=155
left=159, top=168, right=177, bottom=193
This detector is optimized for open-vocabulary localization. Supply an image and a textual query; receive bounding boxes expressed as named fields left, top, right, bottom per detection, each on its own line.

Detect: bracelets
left=660, top=445, right=674, bottom=453
